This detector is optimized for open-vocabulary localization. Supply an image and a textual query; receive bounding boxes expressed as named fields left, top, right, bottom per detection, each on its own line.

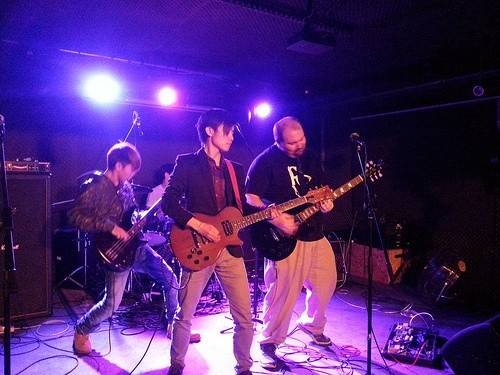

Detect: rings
left=324, top=207, right=327, bottom=211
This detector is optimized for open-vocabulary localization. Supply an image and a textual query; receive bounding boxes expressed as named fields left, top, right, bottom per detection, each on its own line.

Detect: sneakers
left=260, top=344, right=278, bottom=368
left=297, top=319, right=331, bottom=344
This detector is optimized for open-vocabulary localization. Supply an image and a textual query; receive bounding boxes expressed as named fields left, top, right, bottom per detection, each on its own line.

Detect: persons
left=161, top=108, right=283, bottom=375
left=246, top=116, right=337, bottom=368
left=146, top=164, right=175, bottom=209
left=66, top=139, right=201, bottom=354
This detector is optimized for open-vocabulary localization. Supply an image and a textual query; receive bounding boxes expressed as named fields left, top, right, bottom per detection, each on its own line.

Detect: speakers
left=330, top=240, right=346, bottom=282
left=440, top=313, right=500, bottom=375
left=0, top=175, right=52, bottom=322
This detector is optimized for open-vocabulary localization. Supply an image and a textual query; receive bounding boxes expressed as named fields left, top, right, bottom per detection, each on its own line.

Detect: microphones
left=350, top=133, right=367, bottom=147
left=135, top=111, right=143, bottom=136
left=0, top=114, right=5, bottom=137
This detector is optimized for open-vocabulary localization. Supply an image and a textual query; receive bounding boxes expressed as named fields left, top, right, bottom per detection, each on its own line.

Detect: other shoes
left=235, top=365, right=253, bottom=375
left=166, top=365, right=183, bottom=375
left=73, top=329, right=91, bottom=353
left=167, top=324, right=201, bottom=341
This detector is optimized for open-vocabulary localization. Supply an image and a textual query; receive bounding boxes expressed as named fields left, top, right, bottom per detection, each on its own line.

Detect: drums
left=138, top=210, right=171, bottom=248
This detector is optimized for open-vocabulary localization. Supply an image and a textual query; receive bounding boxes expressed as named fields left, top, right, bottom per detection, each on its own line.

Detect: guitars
left=249, top=160, right=384, bottom=261
left=169, top=183, right=337, bottom=270
left=95, top=196, right=165, bottom=272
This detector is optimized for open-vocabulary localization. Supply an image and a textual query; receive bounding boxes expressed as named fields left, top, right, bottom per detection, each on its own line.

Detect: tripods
left=53, top=231, right=98, bottom=290
left=221, top=253, right=264, bottom=334
